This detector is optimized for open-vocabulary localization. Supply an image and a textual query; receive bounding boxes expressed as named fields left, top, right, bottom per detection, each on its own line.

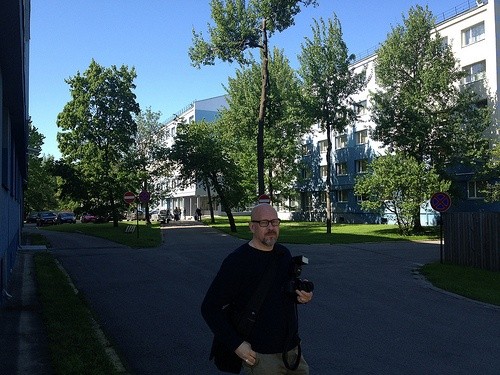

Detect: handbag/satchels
left=209, top=303, right=242, bottom=372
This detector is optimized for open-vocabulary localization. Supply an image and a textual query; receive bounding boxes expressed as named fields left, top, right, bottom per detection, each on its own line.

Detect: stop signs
left=124, top=192, right=136, bottom=204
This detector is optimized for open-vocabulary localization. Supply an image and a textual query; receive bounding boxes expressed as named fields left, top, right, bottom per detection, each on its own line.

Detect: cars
left=81, top=212, right=99, bottom=223
left=26, top=209, right=58, bottom=227
left=57, top=211, right=77, bottom=224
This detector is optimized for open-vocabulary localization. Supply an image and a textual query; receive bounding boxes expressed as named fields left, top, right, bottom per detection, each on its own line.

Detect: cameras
left=285, top=256, right=314, bottom=298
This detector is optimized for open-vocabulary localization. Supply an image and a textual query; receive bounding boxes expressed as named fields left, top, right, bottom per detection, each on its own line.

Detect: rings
left=246, top=359, right=248, bottom=362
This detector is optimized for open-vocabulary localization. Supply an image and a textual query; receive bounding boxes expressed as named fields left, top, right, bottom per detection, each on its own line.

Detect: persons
left=173, top=207, right=186, bottom=220
left=196, top=208, right=202, bottom=221
left=201, top=203, right=313, bottom=375
left=167, top=210, right=170, bottom=218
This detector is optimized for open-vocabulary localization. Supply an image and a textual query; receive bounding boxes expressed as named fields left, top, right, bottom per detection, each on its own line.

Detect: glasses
left=251, top=219, right=282, bottom=227
